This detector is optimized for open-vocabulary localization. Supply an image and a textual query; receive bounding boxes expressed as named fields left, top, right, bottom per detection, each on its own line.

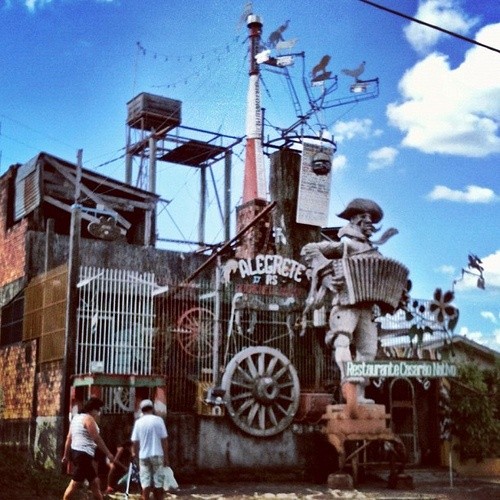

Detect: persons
left=91, top=429, right=124, bottom=496
left=300, top=199, right=412, bottom=403
left=57, top=397, right=115, bottom=500
left=130, top=399, right=167, bottom=500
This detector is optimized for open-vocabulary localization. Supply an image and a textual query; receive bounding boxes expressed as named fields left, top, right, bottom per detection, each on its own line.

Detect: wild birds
left=268, top=19, right=291, bottom=44
left=341, top=61, right=366, bottom=81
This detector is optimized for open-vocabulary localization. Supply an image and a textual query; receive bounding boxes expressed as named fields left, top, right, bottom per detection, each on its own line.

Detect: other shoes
left=105, top=487, right=113, bottom=494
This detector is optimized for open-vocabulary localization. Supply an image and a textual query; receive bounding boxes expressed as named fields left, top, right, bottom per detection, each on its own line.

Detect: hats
left=140, top=399, right=153, bottom=409
